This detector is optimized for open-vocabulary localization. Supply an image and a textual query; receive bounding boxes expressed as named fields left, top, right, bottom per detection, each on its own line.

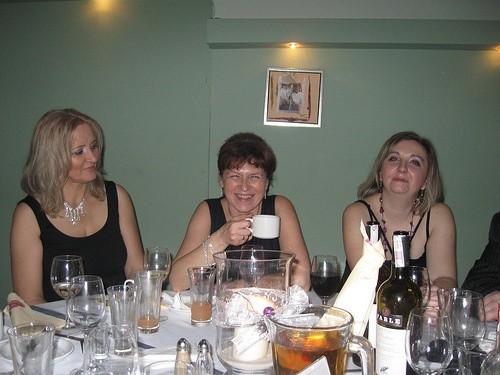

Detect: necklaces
left=379, top=194, right=417, bottom=260
left=63, top=186, right=90, bottom=227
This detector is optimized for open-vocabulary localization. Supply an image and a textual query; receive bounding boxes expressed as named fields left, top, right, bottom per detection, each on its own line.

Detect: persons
left=11, top=109, right=145, bottom=307
left=169, top=132, right=313, bottom=293
left=339, top=130, right=500, bottom=326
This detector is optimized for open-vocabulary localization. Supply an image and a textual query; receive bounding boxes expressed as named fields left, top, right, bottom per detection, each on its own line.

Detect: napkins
left=4, top=292, right=67, bottom=334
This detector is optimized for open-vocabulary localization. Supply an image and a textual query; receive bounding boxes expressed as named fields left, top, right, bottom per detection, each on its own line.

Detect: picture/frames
left=263, top=67, right=324, bottom=129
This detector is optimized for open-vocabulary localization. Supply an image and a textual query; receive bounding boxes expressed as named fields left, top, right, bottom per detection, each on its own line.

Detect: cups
left=82, top=325, right=139, bottom=375
left=262, top=303, right=374, bottom=375
left=244, top=214, right=280, bottom=239
left=133, top=269, right=163, bottom=334
left=187, top=266, right=217, bottom=327
left=107, top=284, right=143, bottom=357
left=7, top=322, right=56, bottom=375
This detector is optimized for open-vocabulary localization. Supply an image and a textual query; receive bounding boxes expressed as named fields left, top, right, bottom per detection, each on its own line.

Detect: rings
left=242, top=235, right=244, bottom=240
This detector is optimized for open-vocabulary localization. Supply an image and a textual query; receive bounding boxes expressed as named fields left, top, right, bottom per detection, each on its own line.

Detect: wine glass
left=50, top=255, right=84, bottom=336
left=310, top=254, right=339, bottom=305
left=143, top=246, right=171, bottom=322
left=66, top=275, right=106, bottom=375
left=238, top=244, right=265, bottom=288
left=403, top=287, right=500, bottom=375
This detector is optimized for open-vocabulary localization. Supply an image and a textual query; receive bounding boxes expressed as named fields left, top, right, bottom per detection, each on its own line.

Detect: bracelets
left=203, top=236, right=217, bottom=265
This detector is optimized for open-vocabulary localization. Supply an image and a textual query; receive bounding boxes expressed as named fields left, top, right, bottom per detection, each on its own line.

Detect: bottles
left=193, top=338, right=214, bottom=375
left=174, top=337, right=190, bottom=375
left=374, top=230, right=423, bottom=375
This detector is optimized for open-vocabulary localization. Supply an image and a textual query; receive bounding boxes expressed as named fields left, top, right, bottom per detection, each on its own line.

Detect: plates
left=0, top=335, right=75, bottom=365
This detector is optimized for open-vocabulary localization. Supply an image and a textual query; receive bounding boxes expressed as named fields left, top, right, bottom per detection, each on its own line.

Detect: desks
left=0, top=288, right=500, bottom=375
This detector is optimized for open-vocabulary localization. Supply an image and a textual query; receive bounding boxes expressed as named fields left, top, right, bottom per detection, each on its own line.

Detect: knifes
left=191, top=362, right=224, bottom=375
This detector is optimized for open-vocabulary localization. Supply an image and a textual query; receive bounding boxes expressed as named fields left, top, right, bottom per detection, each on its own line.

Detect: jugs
left=212, top=249, right=296, bottom=375
